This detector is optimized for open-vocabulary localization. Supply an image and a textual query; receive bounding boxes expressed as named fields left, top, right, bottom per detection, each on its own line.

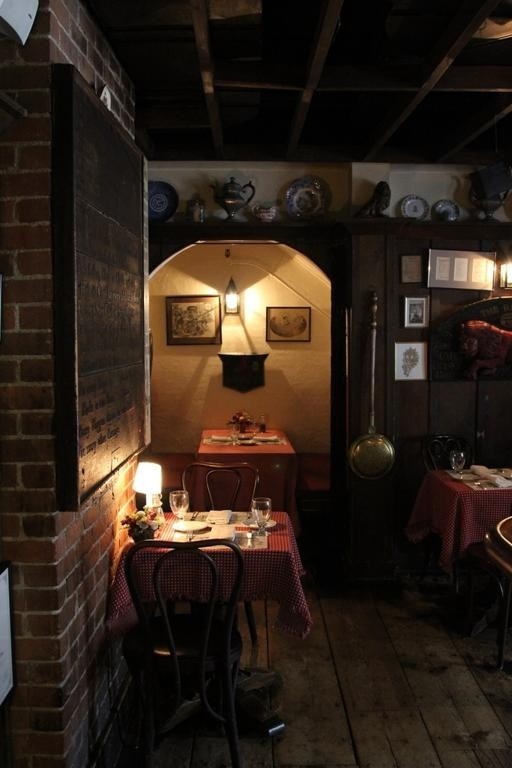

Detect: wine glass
left=169, top=490, right=190, bottom=520
left=251, top=496, right=273, bottom=541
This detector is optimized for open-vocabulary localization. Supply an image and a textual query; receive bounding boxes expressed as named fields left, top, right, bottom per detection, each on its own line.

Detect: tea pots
left=208, top=176, right=255, bottom=220
left=464, top=164, right=511, bottom=222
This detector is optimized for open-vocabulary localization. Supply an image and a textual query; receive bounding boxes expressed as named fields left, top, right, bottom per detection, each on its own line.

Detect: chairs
left=125, top=540, right=244, bottom=767
left=182, top=462, right=259, bottom=511
left=483, top=533, right=512, bottom=671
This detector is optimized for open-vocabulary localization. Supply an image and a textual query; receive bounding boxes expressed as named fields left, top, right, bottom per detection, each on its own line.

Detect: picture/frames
left=266, top=307, right=311, bottom=342
left=392, top=339, right=431, bottom=384
left=166, top=294, right=222, bottom=345
left=403, top=296, right=429, bottom=328
left=400, top=255, right=421, bottom=283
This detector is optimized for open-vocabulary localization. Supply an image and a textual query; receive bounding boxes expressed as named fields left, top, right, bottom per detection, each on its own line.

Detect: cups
left=229, top=424, right=240, bottom=446
left=449, top=451, right=465, bottom=474
left=253, top=203, right=277, bottom=222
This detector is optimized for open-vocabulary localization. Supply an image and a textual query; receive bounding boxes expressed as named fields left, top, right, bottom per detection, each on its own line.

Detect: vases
left=133, top=534, right=154, bottom=543
left=240, top=425, right=246, bottom=432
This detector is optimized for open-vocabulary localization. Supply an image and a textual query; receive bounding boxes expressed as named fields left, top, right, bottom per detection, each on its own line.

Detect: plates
left=450, top=473, right=480, bottom=481
left=286, top=177, right=325, bottom=221
left=241, top=441, right=256, bottom=446
left=242, top=518, right=278, bottom=529
left=147, top=182, right=179, bottom=221
left=429, top=199, right=460, bottom=222
left=399, top=195, right=430, bottom=220
left=173, top=520, right=208, bottom=532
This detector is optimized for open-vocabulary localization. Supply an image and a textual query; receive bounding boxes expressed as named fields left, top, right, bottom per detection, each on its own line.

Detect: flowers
left=121, top=506, right=158, bottom=536
left=226, top=410, right=252, bottom=426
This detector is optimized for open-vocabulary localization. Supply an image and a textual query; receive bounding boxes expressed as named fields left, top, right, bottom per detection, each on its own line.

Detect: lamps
left=224, top=279, right=240, bottom=313
left=133, top=462, right=162, bottom=505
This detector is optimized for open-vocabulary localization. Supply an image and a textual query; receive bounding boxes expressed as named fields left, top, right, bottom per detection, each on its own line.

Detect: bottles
left=187, top=200, right=205, bottom=223
left=259, top=415, right=266, bottom=433
